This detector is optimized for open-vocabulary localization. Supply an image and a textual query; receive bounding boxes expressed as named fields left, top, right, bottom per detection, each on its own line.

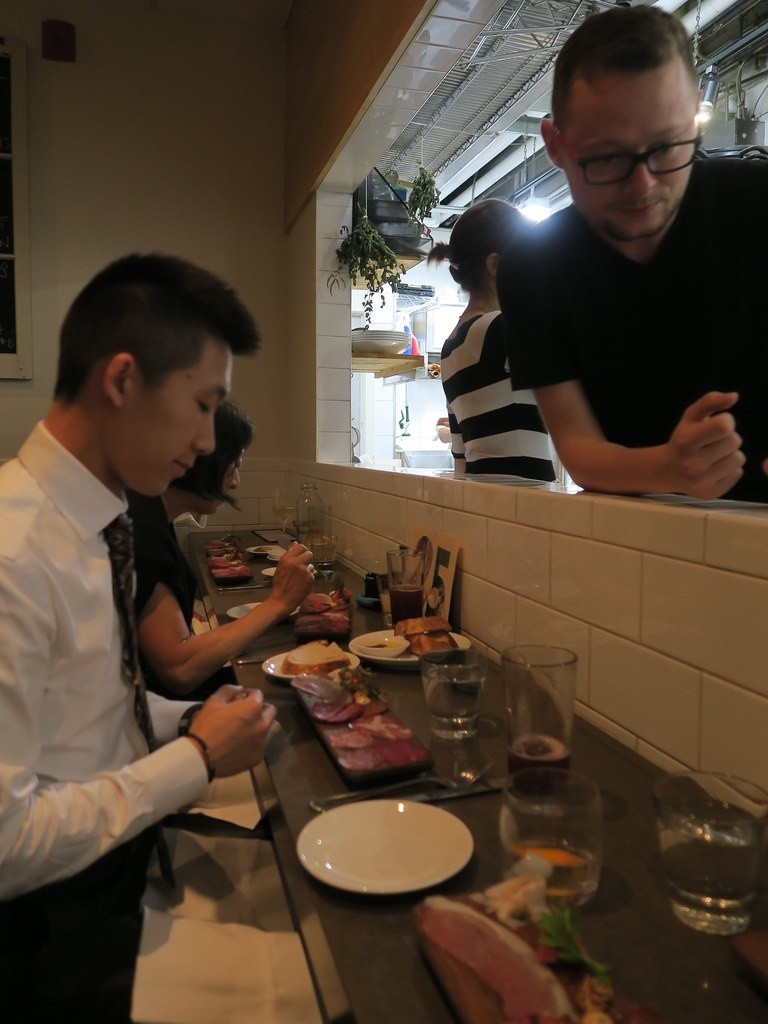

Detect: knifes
left=323, top=784, right=491, bottom=810
left=217, top=581, right=272, bottom=590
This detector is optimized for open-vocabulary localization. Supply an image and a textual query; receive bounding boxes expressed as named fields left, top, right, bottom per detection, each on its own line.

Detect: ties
left=104, top=514, right=178, bottom=904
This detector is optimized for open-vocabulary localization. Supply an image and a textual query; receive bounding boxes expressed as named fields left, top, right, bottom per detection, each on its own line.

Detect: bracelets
left=187, top=734, right=215, bottom=783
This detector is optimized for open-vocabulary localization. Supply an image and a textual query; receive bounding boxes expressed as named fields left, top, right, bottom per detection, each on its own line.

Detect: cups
left=420, top=650, right=488, bottom=739
left=376, top=576, right=394, bottom=627
left=652, top=771, right=768, bottom=936
left=500, top=768, right=604, bottom=912
left=502, top=644, right=579, bottom=764
left=304, top=506, right=338, bottom=566
left=386, top=549, right=425, bottom=628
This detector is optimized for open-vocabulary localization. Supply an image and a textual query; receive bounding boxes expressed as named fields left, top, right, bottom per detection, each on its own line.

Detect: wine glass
left=271, top=491, right=298, bottom=546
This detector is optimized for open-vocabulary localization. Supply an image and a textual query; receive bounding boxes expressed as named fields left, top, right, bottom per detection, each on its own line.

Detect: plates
left=227, top=603, right=301, bottom=619
left=262, top=650, right=360, bottom=683
left=261, top=567, right=318, bottom=576
left=267, top=555, right=280, bottom=561
left=296, top=799, right=473, bottom=895
left=349, top=629, right=471, bottom=668
left=351, top=330, right=411, bottom=355
left=245, top=545, right=282, bottom=553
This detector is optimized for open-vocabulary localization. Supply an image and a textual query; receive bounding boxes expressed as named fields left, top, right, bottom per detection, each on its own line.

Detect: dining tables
left=191, top=528, right=768, bottom=1024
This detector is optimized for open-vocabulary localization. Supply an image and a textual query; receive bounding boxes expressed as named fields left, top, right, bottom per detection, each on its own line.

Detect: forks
left=293, top=542, right=320, bottom=580
left=316, top=759, right=494, bottom=800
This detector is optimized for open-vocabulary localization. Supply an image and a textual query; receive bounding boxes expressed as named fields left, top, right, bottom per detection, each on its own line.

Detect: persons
left=428, top=199, right=561, bottom=481
left=127, top=405, right=315, bottom=696
left=0, top=253, right=276, bottom=1024
left=495, top=5, right=767, bottom=503
left=436, top=418, right=452, bottom=442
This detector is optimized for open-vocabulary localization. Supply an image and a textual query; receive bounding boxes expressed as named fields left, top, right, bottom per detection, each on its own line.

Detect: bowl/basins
left=360, top=635, right=409, bottom=657
left=268, top=550, right=287, bottom=559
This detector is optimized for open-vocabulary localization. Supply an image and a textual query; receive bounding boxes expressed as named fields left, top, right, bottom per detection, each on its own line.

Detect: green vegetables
left=540, top=904, right=613, bottom=980
left=338, top=665, right=381, bottom=696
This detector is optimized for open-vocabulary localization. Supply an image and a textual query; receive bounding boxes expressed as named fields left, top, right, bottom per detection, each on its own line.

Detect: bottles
left=299, top=481, right=323, bottom=540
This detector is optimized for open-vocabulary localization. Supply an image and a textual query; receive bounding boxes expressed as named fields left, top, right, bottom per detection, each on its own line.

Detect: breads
left=395, top=616, right=459, bottom=653
left=281, top=640, right=352, bottom=676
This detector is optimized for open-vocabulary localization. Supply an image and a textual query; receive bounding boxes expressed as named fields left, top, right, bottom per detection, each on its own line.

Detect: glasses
left=577, top=135, right=702, bottom=187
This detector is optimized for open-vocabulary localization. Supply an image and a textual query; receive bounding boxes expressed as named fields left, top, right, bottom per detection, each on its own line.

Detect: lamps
left=699, top=80, right=719, bottom=120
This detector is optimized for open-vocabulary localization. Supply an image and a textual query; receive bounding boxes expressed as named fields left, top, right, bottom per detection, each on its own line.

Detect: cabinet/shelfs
left=350, top=167, right=433, bottom=378
